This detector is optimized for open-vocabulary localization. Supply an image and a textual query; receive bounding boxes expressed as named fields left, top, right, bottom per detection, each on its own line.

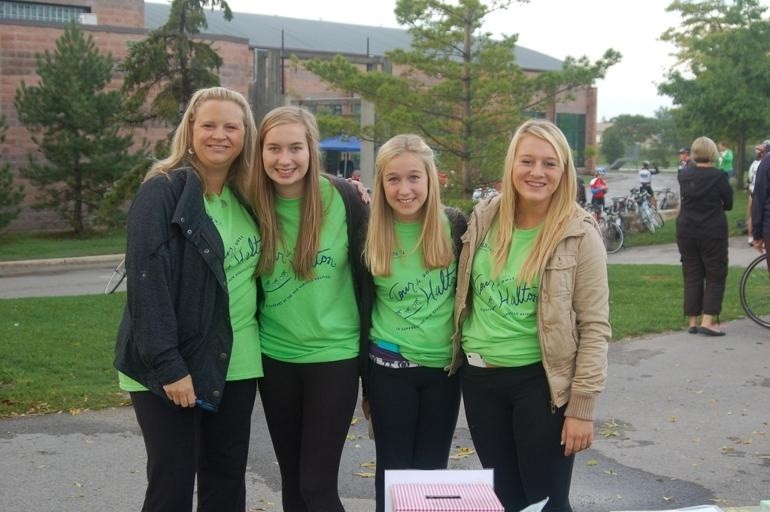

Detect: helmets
left=595, top=168, right=606, bottom=177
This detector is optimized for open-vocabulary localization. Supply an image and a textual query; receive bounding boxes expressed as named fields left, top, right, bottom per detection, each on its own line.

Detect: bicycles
left=740, top=239, right=770, bottom=329
left=579, top=186, right=679, bottom=256
left=103, top=257, right=126, bottom=296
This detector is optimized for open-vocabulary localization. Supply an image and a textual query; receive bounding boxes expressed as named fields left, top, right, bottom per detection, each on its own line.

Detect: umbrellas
left=318, top=132, right=365, bottom=178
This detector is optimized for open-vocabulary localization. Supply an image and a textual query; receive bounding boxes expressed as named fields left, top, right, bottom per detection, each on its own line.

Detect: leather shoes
left=688, top=326, right=725, bottom=336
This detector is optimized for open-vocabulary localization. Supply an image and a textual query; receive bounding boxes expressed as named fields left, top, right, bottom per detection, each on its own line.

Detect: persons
left=444, top=116, right=611, bottom=512
left=638, top=160, right=656, bottom=212
left=338, top=152, right=354, bottom=179
left=677, top=136, right=770, bottom=337
left=251, top=105, right=372, bottom=512
left=346, top=134, right=467, bottom=512
left=112, top=87, right=372, bottom=512
left=468, top=162, right=608, bottom=224
